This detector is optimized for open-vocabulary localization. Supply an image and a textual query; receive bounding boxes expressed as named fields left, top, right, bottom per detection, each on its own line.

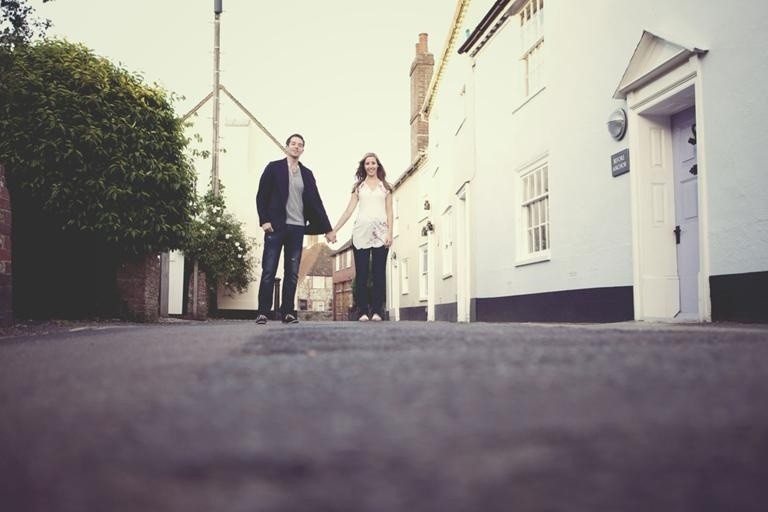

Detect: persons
left=256, top=134, right=396, bottom=325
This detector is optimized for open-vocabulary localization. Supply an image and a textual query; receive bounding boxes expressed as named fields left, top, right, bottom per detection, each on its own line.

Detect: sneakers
left=359, top=313, right=382, bottom=322
left=256, top=315, right=268, bottom=324
left=282, top=313, right=298, bottom=323
